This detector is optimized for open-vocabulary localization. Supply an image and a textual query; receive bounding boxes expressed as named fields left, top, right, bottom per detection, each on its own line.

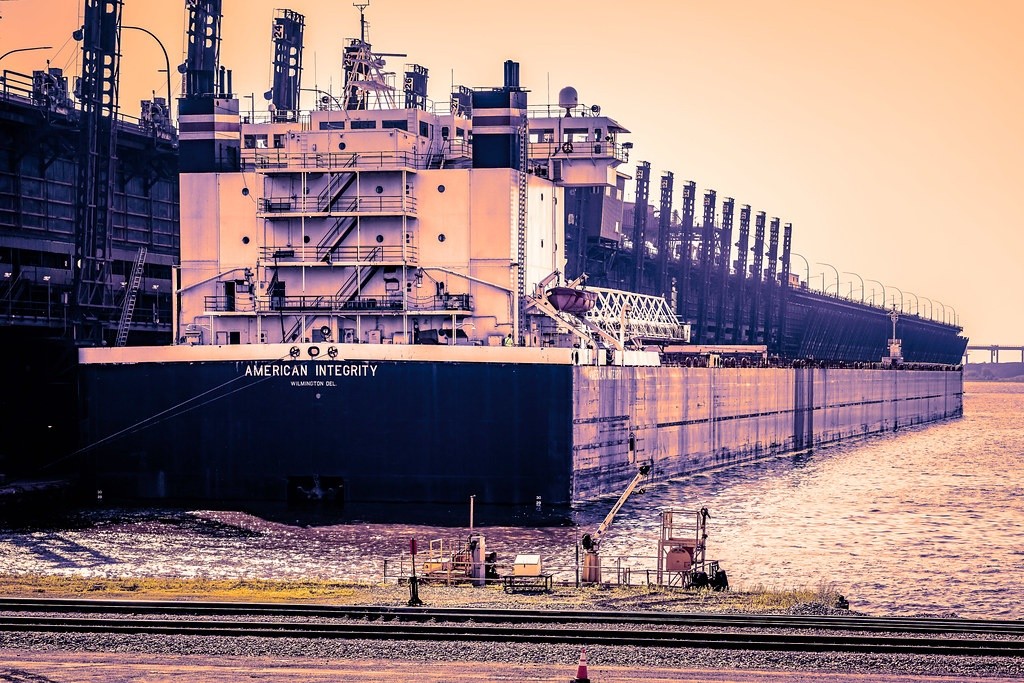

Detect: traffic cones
left=569, top=646, right=591, bottom=683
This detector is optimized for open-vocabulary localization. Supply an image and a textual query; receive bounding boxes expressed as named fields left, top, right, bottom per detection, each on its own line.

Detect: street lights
left=905, top=292, right=919, bottom=316
left=936, top=301, right=945, bottom=324
left=242, top=96, right=255, bottom=125
left=946, top=305, right=956, bottom=326
left=843, top=272, right=864, bottom=302
left=866, top=280, right=885, bottom=309
left=803, top=276, right=820, bottom=291
left=817, top=263, right=839, bottom=296
left=921, top=296, right=932, bottom=321
left=825, top=283, right=836, bottom=295
left=887, top=286, right=903, bottom=312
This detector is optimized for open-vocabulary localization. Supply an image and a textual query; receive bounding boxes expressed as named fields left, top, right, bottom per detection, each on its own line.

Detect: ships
left=76, top=0, right=967, bottom=512
